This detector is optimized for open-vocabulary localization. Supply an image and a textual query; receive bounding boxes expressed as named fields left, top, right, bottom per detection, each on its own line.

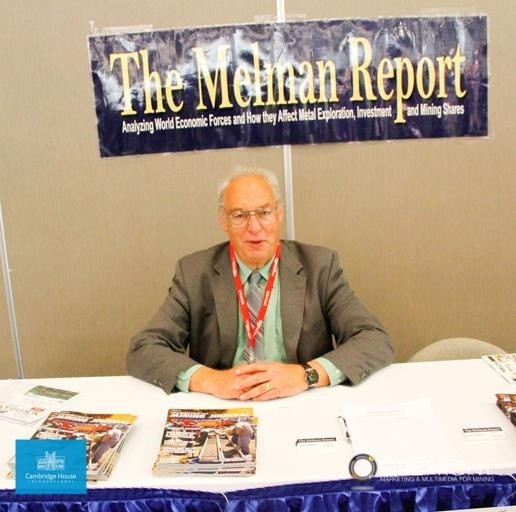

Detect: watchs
left=302, top=362, right=320, bottom=390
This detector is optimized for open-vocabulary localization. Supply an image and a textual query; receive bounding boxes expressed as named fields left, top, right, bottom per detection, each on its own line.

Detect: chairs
left=408, top=336, right=511, bottom=364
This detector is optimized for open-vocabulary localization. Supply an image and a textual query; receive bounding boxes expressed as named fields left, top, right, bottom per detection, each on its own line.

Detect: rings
left=266, top=382, right=272, bottom=390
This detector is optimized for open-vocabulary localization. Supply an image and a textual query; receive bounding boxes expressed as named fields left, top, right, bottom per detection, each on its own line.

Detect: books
left=1, top=386, right=80, bottom=425
left=497, top=394, right=515, bottom=427
left=151, top=408, right=260, bottom=476
left=8, top=411, right=138, bottom=480
left=482, top=354, right=516, bottom=383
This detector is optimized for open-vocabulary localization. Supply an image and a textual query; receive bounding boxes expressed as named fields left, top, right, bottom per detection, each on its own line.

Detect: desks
left=1, top=354, right=515, bottom=511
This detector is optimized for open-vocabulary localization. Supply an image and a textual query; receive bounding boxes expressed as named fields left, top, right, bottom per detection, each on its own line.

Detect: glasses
left=223, top=204, right=279, bottom=227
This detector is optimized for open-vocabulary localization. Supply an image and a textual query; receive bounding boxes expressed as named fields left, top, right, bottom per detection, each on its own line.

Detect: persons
left=126, top=165, right=395, bottom=401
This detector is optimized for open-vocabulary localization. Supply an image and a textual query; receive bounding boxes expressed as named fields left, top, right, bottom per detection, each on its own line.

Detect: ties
left=245, top=273, right=264, bottom=361
